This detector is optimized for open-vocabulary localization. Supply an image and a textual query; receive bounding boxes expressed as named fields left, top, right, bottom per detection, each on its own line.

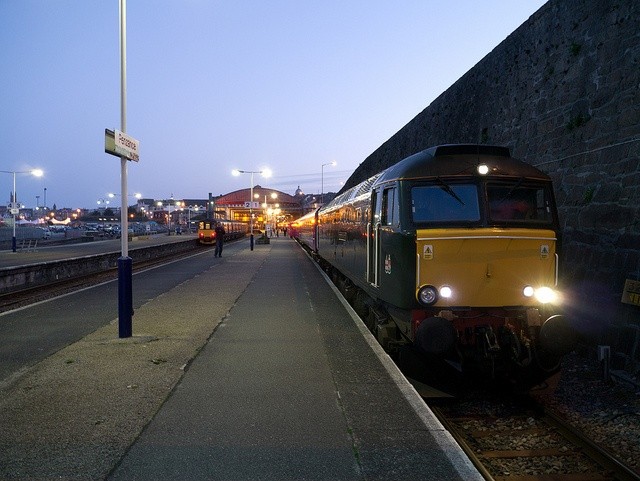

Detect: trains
left=198, top=217, right=248, bottom=244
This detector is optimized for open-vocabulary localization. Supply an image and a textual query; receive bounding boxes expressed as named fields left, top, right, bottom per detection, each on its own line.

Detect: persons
left=212, top=222, right=225, bottom=258
left=266, top=223, right=290, bottom=240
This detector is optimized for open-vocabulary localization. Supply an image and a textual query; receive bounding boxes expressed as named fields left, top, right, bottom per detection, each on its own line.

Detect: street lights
left=262, top=201, right=279, bottom=221
left=254, top=191, right=277, bottom=222
left=286, top=143, right=580, bottom=363
left=156, top=200, right=181, bottom=235
left=0, top=168, right=43, bottom=251
left=109, top=192, right=141, bottom=240
left=232, top=167, right=272, bottom=250
left=322, top=159, right=336, bottom=202
